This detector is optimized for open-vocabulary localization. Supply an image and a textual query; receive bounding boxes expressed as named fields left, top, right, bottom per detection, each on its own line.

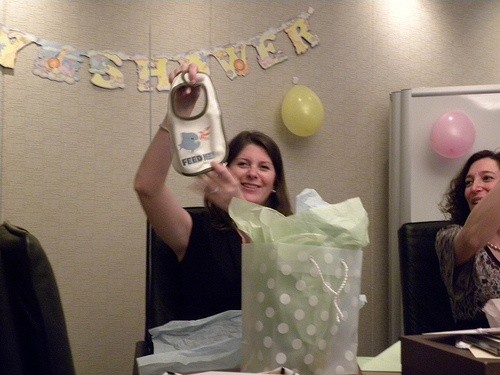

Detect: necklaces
left=486, top=240, right=500, bottom=251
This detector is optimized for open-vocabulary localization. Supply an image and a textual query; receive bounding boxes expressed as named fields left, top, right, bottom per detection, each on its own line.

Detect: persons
left=134, top=64, right=295, bottom=321
left=435, top=149, right=500, bottom=333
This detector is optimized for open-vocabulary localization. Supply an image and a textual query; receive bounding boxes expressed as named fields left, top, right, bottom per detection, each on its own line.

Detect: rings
left=211, top=187, right=220, bottom=195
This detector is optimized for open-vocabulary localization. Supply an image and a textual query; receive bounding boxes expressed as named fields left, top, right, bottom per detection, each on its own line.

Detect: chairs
left=0, top=221, right=77, bottom=375
left=397, top=220, right=461, bottom=335
left=145, top=207, right=206, bottom=338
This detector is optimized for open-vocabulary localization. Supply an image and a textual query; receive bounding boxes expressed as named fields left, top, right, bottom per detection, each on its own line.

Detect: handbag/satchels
left=241, top=242, right=365, bottom=374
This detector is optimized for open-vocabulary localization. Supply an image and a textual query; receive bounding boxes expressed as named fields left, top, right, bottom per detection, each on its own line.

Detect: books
left=422, top=327, right=500, bottom=356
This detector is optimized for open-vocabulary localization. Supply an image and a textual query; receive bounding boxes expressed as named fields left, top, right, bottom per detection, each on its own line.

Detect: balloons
left=281, top=84, right=323, bottom=137
left=431, top=110, right=477, bottom=159
